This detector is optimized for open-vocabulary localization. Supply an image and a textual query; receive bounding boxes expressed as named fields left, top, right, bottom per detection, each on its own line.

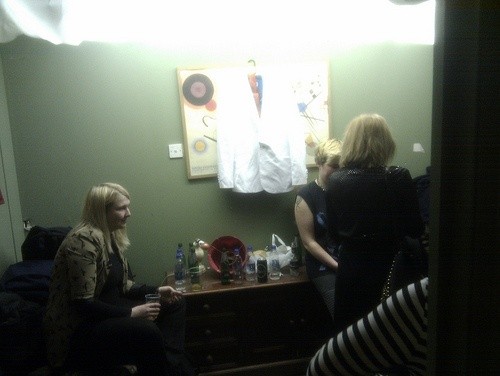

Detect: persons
left=43, top=182, right=196, bottom=376
left=306, top=276, right=428, bottom=376
left=326, top=113, right=425, bottom=333
left=295, top=138, right=345, bottom=322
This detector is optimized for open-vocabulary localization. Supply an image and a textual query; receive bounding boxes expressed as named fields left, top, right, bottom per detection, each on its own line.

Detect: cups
left=145, top=294, right=161, bottom=320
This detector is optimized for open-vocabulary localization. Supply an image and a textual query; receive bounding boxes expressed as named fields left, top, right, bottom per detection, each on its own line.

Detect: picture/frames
left=176, top=64, right=331, bottom=180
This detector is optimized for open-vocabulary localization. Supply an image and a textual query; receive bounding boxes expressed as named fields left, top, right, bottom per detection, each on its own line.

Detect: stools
left=29, top=363, right=137, bottom=376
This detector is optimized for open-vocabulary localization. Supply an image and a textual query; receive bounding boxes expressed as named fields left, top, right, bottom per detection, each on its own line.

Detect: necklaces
left=316, top=177, right=326, bottom=191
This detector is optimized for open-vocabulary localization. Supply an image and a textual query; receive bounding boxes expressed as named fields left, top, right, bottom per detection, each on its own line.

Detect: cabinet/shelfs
left=173, top=266, right=318, bottom=371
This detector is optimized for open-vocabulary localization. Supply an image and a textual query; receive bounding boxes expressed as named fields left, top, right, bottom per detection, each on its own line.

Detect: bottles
left=269, top=246, right=280, bottom=280
left=188, top=267, right=206, bottom=292
left=232, top=249, right=243, bottom=283
left=188, top=242, right=198, bottom=269
left=246, top=246, right=256, bottom=281
left=289, top=242, right=299, bottom=276
left=221, top=249, right=230, bottom=284
left=175, top=242, right=186, bottom=285
